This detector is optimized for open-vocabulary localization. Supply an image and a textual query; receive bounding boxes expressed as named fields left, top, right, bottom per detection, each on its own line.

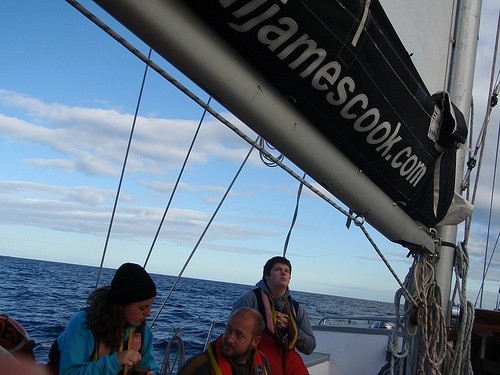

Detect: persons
left=42, top=263, right=159, bottom=375
left=176, top=306, right=272, bottom=375
left=0, top=313, right=38, bottom=364
left=0, top=345, right=51, bottom=375
left=230, top=256, right=316, bottom=375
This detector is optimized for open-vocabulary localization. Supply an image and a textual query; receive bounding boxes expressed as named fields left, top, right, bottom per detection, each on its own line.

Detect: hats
left=110, top=262, right=157, bottom=304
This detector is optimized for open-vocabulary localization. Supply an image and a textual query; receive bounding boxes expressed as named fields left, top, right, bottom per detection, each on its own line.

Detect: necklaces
left=268, top=296, right=299, bottom=352
left=119, top=321, right=133, bottom=375
